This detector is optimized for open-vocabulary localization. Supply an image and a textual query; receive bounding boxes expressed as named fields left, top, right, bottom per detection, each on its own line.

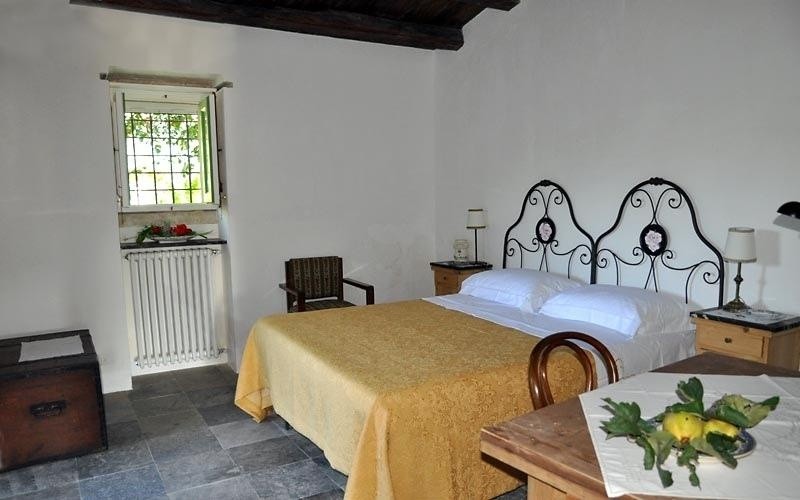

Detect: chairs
left=279, top=256, right=374, bottom=313
left=527, top=331, right=620, bottom=409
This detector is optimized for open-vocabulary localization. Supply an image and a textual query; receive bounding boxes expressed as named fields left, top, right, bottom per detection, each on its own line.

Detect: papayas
left=661, top=412, right=703, bottom=444
left=703, top=415, right=740, bottom=439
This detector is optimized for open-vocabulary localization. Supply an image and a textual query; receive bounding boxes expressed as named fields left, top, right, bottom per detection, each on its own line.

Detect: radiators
left=125, top=247, right=225, bottom=368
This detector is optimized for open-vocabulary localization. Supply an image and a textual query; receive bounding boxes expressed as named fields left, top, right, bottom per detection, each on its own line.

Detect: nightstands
left=430, top=261, right=487, bottom=296
left=689, top=306, right=800, bottom=371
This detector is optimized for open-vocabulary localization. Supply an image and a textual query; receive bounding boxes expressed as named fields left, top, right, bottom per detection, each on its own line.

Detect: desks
left=479, top=351, right=799, bottom=499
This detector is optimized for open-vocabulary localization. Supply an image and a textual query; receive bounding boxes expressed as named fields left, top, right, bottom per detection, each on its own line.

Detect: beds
left=259, top=176, right=725, bottom=500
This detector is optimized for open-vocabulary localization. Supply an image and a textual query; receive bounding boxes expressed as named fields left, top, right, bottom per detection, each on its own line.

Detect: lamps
left=466, top=209, right=487, bottom=265
left=723, top=227, right=757, bottom=312
left=777, top=202, right=800, bottom=220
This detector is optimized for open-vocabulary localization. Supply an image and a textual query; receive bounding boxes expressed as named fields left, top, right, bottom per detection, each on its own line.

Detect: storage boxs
left=1, top=329, right=108, bottom=475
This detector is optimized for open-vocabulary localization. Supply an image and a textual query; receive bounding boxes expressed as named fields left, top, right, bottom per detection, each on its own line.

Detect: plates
left=652, top=421, right=757, bottom=459
left=150, top=235, right=193, bottom=244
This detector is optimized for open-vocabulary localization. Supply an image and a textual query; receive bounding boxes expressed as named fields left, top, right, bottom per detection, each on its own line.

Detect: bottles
left=452, top=239, right=469, bottom=264
